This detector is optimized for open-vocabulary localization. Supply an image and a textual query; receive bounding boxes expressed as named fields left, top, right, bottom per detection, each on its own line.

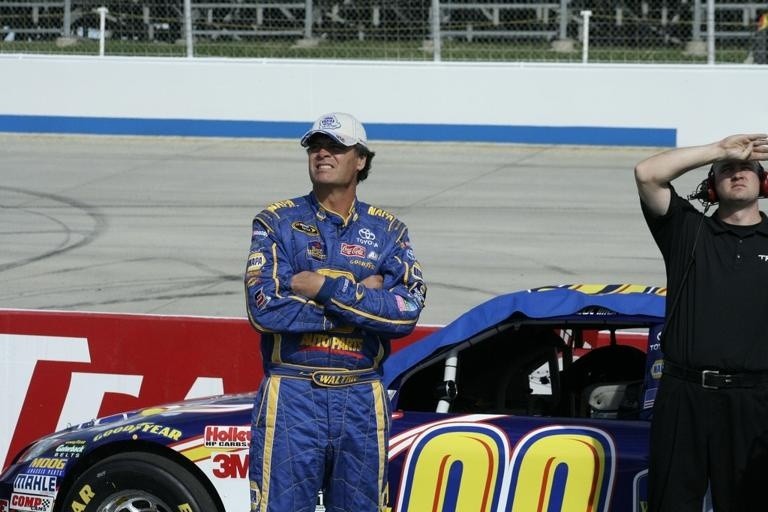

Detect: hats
left=301, top=111, right=368, bottom=150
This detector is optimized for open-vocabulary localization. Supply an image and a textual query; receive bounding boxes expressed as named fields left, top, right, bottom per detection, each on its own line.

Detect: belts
left=663, top=361, right=768, bottom=390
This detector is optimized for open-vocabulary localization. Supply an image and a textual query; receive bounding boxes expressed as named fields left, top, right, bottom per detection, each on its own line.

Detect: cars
left=1, top=284, right=666, bottom=512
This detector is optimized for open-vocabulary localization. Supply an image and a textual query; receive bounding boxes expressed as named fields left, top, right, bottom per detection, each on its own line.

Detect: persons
left=632, top=132, right=767, bottom=510
left=243, top=113, right=428, bottom=512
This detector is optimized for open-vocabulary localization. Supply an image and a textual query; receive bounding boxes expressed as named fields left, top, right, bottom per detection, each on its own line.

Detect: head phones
left=689, top=163, right=768, bottom=208
